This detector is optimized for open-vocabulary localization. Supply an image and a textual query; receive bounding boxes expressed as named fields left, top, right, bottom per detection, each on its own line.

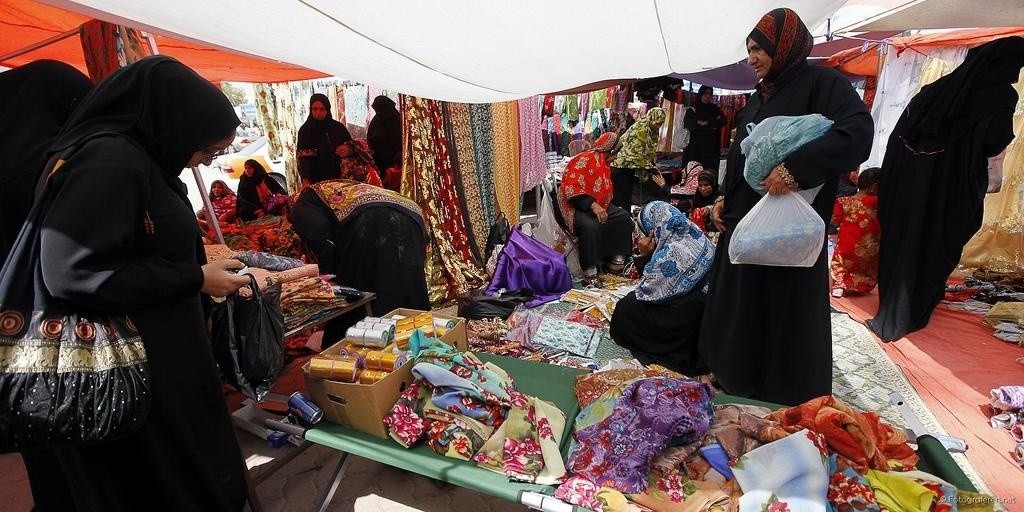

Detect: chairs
left=666, top=193, right=696, bottom=213
left=542, top=175, right=580, bottom=258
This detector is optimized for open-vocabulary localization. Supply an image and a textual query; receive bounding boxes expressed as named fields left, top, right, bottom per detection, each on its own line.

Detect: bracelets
left=777, top=162, right=802, bottom=193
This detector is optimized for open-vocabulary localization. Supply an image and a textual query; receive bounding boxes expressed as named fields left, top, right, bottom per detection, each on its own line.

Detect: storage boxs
left=298, top=305, right=470, bottom=440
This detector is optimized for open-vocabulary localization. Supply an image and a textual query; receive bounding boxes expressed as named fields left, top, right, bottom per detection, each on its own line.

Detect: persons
left=830, top=166, right=882, bottom=299
left=866, top=36, right=1024, bottom=343
left=694, top=10, right=876, bottom=406
left=15, top=52, right=251, bottom=512
left=194, top=84, right=728, bottom=378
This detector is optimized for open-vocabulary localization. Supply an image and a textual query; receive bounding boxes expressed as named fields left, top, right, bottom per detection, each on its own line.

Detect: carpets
left=831, top=309, right=1008, bottom=512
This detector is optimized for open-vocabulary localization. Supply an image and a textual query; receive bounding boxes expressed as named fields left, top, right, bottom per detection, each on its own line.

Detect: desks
left=280, top=285, right=377, bottom=363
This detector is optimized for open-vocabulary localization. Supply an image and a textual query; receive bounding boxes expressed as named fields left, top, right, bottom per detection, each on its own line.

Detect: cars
left=215, top=132, right=287, bottom=194
left=222, top=134, right=254, bottom=154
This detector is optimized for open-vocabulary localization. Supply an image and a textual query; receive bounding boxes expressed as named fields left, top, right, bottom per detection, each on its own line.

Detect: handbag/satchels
left=1, top=254, right=153, bottom=452
